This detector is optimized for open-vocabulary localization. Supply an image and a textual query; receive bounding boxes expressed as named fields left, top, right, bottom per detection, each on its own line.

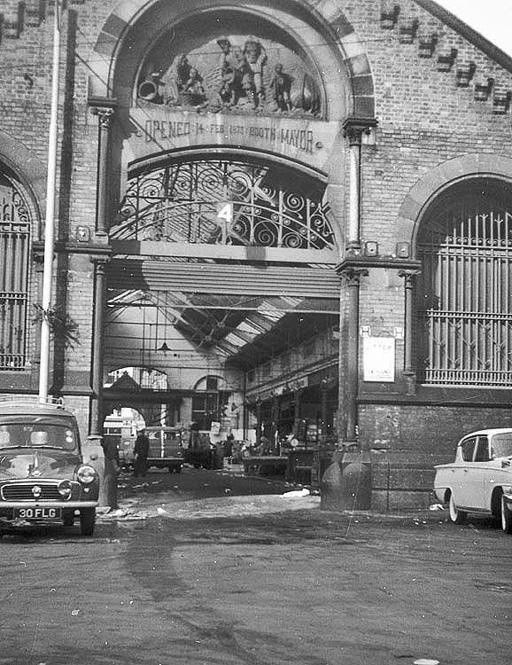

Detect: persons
left=132, top=430, right=149, bottom=478
left=179, top=38, right=293, bottom=114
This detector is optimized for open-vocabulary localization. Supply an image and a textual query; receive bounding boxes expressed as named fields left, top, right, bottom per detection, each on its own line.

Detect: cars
left=216, top=440, right=242, bottom=457
left=117, top=439, right=184, bottom=473
left=433, top=428, right=512, bottom=534
left=0, top=394, right=99, bottom=539
